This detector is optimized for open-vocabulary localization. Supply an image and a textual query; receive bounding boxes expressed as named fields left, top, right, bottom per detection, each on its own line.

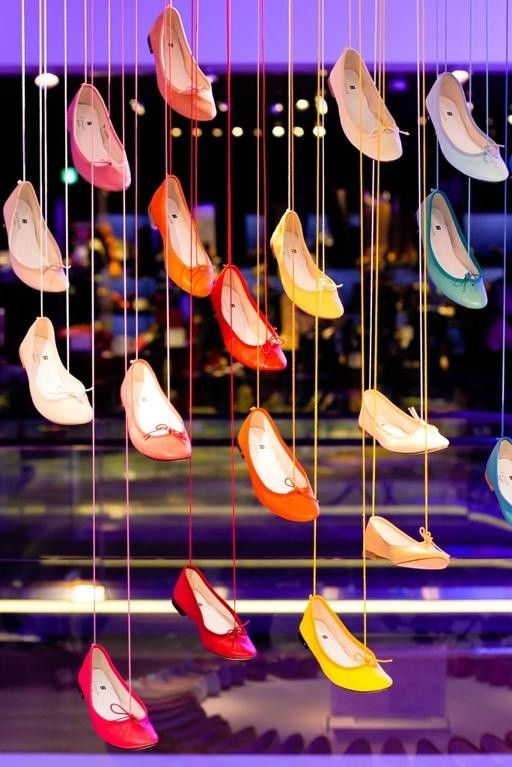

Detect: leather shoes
left=418, top=191, right=489, bottom=309
left=76, top=644, right=160, bottom=752
left=425, top=71, right=509, bottom=183
left=67, top=84, right=133, bottom=193
left=148, top=175, right=215, bottom=299
left=269, top=209, right=344, bottom=320
left=264, top=647, right=319, bottom=679
left=171, top=564, right=258, bottom=662
left=18, top=317, right=94, bottom=426
left=210, top=265, right=288, bottom=374
left=297, top=594, right=394, bottom=693
left=485, top=438, right=512, bottom=528
left=237, top=408, right=321, bottom=524
left=3, top=181, right=71, bottom=293
left=147, top=5, right=218, bottom=121
left=107, top=650, right=280, bottom=754
left=448, top=648, right=512, bottom=688
left=362, top=515, right=448, bottom=570
left=328, top=48, right=410, bottom=163
left=121, top=359, right=192, bottom=461
left=358, top=389, right=449, bottom=455
